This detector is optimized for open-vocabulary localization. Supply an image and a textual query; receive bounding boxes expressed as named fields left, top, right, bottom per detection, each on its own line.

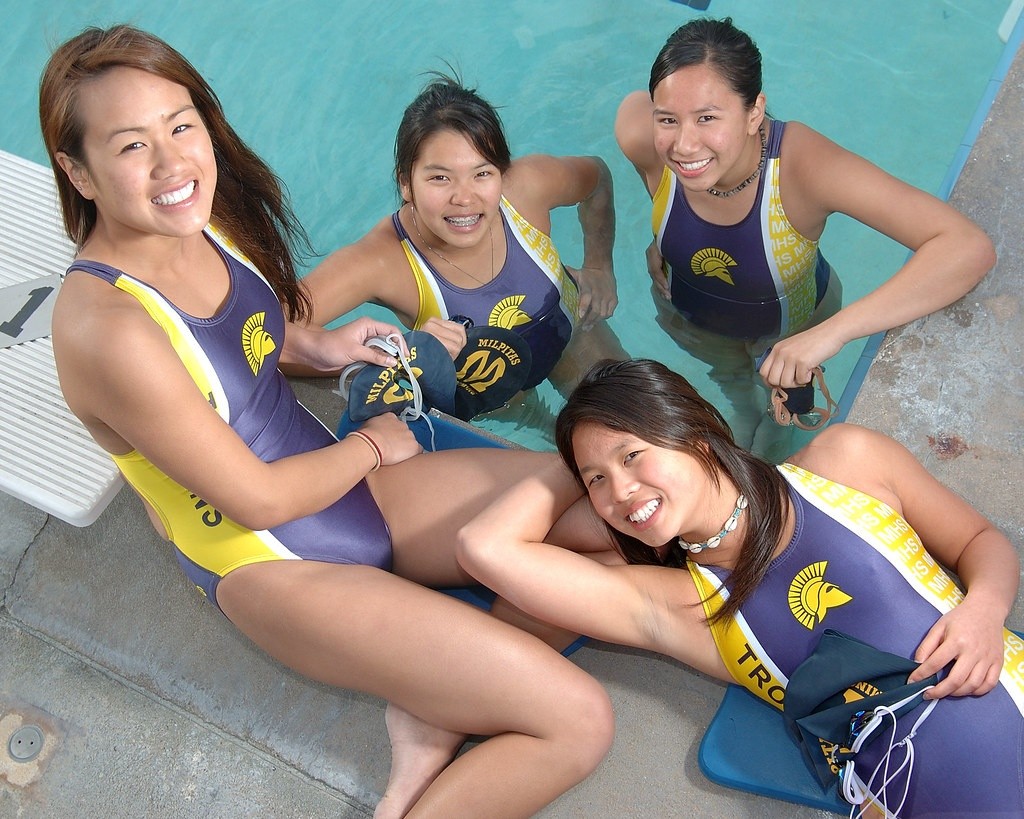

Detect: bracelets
left=346, top=431, right=382, bottom=472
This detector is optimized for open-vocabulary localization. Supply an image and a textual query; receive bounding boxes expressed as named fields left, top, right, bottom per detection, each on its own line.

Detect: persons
left=612, top=18, right=998, bottom=388
left=277, top=84, right=599, bottom=377
left=456, top=357, right=1023, bottom=819
left=40, top=26, right=668, bottom=819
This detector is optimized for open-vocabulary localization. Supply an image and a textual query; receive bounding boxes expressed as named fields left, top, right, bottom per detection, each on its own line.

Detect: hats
left=453, top=326, right=533, bottom=422
left=782, top=629, right=939, bottom=788
left=348, top=330, right=457, bottom=422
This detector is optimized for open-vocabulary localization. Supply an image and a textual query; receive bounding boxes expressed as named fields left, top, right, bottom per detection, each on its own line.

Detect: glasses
left=837, top=706, right=884, bottom=804
left=766, top=397, right=831, bottom=430
left=449, top=316, right=473, bottom=329
left=338, top=335, right=398, bottom=401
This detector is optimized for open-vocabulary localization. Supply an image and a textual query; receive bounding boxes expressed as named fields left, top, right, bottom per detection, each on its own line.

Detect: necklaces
left=706, top=125, right=767, bottom=198
left=411, top=205, right=494, bottom=285
left=679, top=493, right=748, bottom=554
